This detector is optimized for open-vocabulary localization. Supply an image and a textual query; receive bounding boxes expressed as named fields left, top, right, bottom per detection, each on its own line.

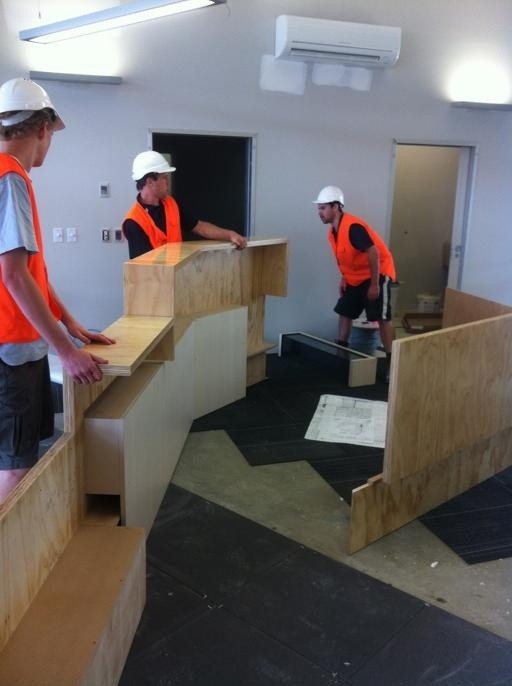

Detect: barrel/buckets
left=416, top=294, right=441, bottom=314
left=352, top=321, right=380, bottom=355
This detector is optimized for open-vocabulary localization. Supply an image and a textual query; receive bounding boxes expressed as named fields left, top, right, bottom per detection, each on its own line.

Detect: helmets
left=131, top=150, right=177, bottom=181
left=0, top=77, right=66, bottom=131
left=312, top=185, right=344, bottom=208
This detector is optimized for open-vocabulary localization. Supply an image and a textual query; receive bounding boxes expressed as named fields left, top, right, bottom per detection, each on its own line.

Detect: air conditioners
left=275, top=15, right=402, bottom=69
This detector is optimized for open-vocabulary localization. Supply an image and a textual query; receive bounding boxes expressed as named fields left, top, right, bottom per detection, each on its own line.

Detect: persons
left=120, top=149, right=249, bottom=260
left=311, top=185, right=398, bottom=386
left=0, top=74, right=118, bottom=506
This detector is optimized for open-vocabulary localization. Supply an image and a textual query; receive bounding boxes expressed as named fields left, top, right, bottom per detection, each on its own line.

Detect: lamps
left=29, top=70, right=121, bottom=86
left=451, top=100, right=511, bottom=111
left=19, top=0, right=226, bottom=44
